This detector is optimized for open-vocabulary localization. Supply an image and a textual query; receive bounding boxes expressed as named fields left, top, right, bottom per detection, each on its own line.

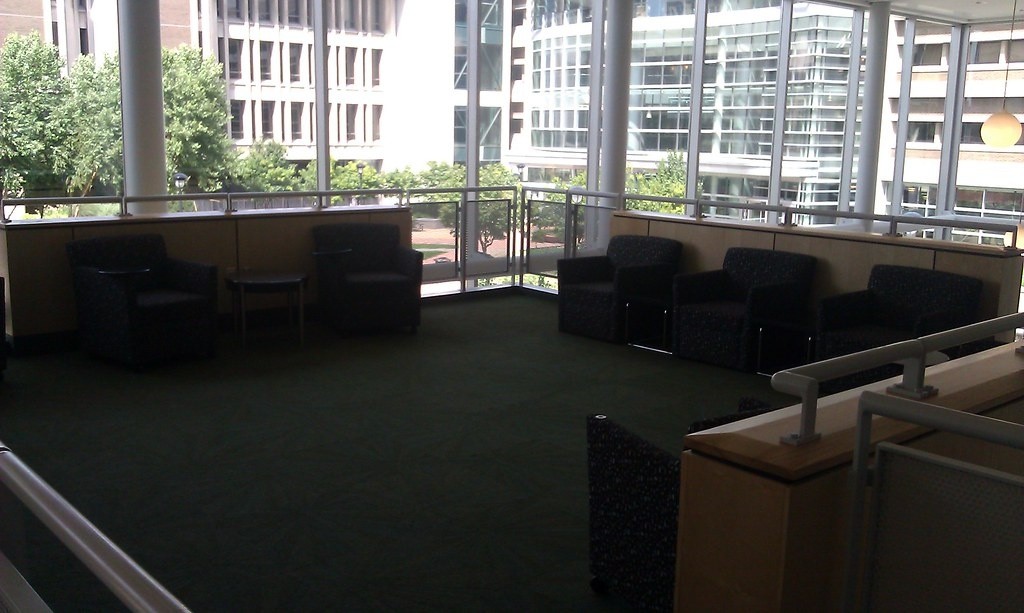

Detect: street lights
left=174, top=173, right=187, bottom=213
left=570, top=187, right=585, bottom=258
left=357, top=163, right=366, bottom=205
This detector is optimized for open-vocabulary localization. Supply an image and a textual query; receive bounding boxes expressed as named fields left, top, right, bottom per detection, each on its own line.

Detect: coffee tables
left=226, top=268, right=309, bottom=351
left=624, top=288, right=672, bottom=357
left=756, top=312, right=813, bottom=378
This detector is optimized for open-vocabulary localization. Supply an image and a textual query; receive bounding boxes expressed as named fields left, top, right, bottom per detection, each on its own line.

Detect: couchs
left=556, top=234, right=683, bottom=345
left=312, top=223, right=422, bottom=339
left=587, top=396, right=770, bottom=611
left=673, top=247, right=820, bottom=373
left=66, top=235, right=221, bottom=375
left=816, top=265, right=1004, bottom=395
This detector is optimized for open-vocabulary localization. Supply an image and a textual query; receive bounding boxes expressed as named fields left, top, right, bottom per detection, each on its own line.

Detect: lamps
left=980, top=0, right=1022, bottom=149
